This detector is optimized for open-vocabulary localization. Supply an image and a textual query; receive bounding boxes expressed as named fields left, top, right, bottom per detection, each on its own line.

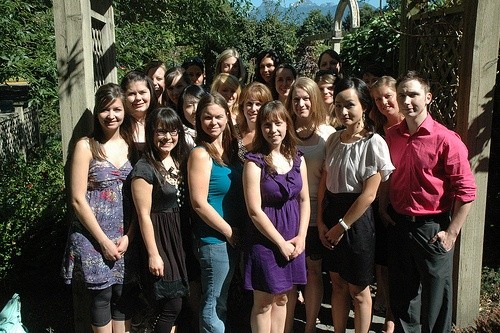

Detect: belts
left=394, top=213, right=447, bottom=223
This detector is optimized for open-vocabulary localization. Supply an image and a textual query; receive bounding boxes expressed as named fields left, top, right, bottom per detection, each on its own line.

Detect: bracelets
left=339, top=218, right=351, bottom=232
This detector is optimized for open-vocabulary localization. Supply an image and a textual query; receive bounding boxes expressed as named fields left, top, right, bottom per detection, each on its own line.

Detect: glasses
left=183, top=58, right=203, bottom=64
left=316, top=69, right=339, bottom=80
left=155, top=129, right=180, bottom=136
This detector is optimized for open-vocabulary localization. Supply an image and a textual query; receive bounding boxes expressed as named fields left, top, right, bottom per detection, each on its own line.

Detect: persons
left=359, top=68, right=406, bottom=333
left=66, top=82, right=138, bottom=333
left=315, top=75, right=384, bottom=333
left=379, top=74, right=477, bottom=333
left=120, top=46, right=344, bottom=333
